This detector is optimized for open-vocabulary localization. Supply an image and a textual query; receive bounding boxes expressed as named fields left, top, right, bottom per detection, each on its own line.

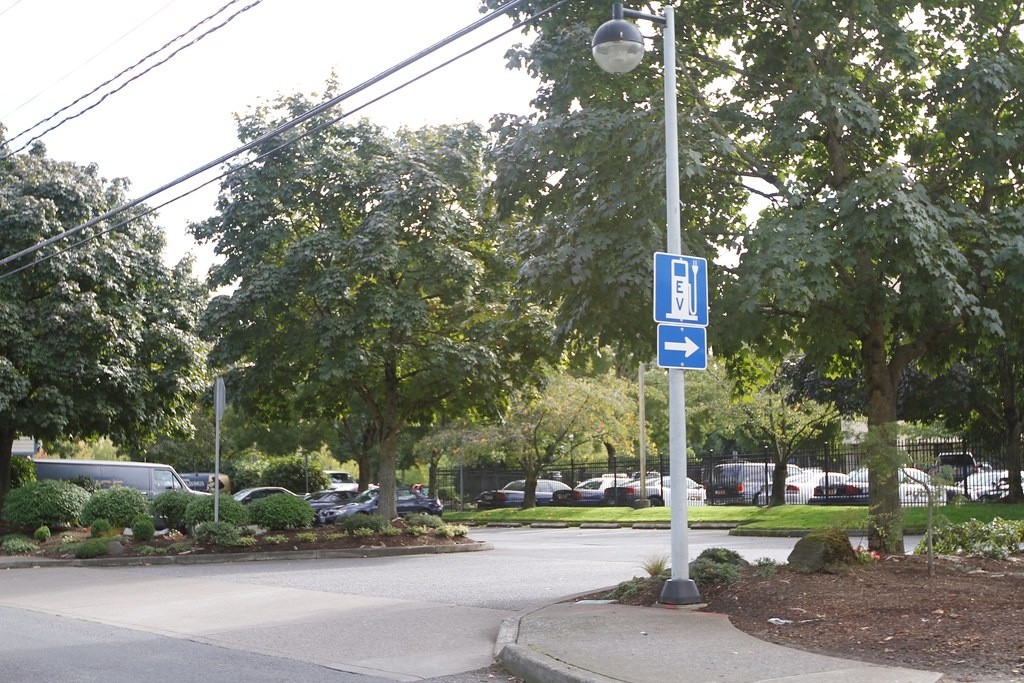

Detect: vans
left=704, top=463, right=803, bottom=505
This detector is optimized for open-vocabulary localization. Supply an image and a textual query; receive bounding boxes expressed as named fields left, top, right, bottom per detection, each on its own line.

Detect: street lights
left=592, top=2, right=702, bottom=606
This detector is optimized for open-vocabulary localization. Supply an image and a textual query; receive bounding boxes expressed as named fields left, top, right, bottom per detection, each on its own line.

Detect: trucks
left=32, top=459, right=212, bottom=528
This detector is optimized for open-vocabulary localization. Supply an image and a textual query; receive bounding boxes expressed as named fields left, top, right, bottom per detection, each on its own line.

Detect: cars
left=323, top=471, right=359, bottom=492
left=947, top=470, right=1024, bottom=505
left=552, top=477, right=633, bottom=506
left=317, top=487, right=443, bottom=523
left=928, top=452, right=979, bottom=487
left=305, top=491, right=371, bottom=513
left=758, top=473, right=851, bottom=504
left=808, top=467, right=978, bottom=507
left=232, top=487, right=306, bottom=505
left=604, top=475, right=707, bottom=506
left=476, top=480, right=573, bottom=510
